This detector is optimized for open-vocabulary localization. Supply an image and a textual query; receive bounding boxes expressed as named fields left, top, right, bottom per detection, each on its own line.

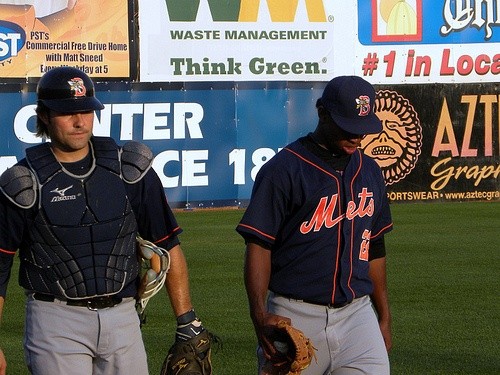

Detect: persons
left=0, top=66, right=214, bottom=375
left=234, top=75, right=392, bottom=375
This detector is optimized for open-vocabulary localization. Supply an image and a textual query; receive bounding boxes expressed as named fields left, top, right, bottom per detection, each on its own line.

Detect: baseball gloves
left=262, top=315, right=316, bottom=375
left=160, top=329, right=213, bottom=375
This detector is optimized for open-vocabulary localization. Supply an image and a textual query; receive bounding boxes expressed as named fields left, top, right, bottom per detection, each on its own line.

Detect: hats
left=322, top=75, right=383, bottom=134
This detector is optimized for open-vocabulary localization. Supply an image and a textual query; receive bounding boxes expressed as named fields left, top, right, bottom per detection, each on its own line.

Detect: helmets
left=37, top=67, right=105, bottom=113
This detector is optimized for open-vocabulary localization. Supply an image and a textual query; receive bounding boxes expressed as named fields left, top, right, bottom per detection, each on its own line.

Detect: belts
left=26, top=290, right=123, bottom=310
left=269, top=290, right=352, bottom=310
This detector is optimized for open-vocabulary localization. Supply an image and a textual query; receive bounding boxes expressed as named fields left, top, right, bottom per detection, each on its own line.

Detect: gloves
left=176, top=311, right=208, bottom=360
